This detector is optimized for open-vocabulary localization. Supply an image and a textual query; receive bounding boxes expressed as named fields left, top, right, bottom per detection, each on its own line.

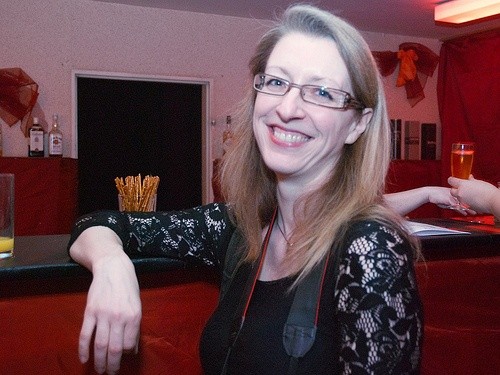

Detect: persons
left=448, top=175, right=500, bottom=219
left=383, top=185, right=477, bottom=217
left=68, top=5, right=424, bottom=375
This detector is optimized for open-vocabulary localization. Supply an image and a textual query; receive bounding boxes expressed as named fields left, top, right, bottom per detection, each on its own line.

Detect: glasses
left=253, top=73, right=365, bottom=111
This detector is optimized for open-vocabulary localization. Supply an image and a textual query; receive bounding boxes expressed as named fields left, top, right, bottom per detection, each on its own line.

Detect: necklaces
left=276, top=220, right=295, bottom=247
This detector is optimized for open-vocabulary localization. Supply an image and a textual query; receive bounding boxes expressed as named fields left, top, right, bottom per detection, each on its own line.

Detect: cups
left=0, top=173, right=15, bottom=259
left=493, top=182, right=500, bottom=227
left=119, top=193, right=156, bottom=213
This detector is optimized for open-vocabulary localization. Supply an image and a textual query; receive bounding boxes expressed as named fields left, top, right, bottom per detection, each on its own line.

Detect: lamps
left=434, top=0, right=500, bottom=24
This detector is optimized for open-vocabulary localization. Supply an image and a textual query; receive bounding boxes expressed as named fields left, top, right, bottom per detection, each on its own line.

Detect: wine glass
left=450, top=142, right=474, bottom=210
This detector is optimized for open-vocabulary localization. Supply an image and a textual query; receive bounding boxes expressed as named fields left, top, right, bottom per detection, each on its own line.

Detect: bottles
left=48, top=113, right=63, bottom=157
left=27, top=117, right=45, bottom=158
left=223, top=115, right=234, bottom=156
